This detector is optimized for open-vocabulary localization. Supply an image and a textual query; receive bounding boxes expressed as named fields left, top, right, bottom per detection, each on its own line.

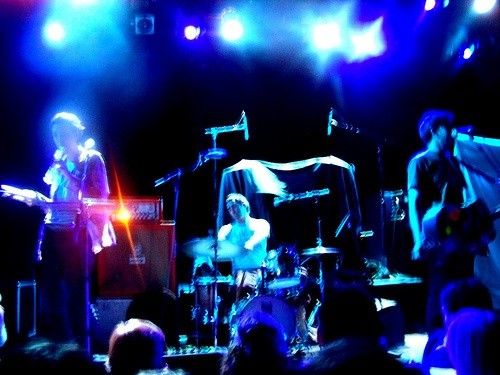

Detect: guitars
left=419, top=196, right=500, bottom=255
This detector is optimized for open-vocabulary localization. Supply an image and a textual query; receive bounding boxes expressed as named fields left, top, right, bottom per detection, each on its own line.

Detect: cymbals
left=181, top=229, right=240, bottom=261
left=299, top=241, right=342, bottom=256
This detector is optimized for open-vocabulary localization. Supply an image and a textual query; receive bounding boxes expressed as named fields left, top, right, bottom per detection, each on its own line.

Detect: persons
left=266, top=253, right=312, bottom=346
left=406, top=111, right=467, bottom=339
left=359, top=241, right=406, bottom=358
left=37, top=112, right=109, bottom=344
left=216, top=192, right=271, bottom=302
left=1, top=279, right=500, bottom=375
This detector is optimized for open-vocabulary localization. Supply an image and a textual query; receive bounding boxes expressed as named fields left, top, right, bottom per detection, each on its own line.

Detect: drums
left=194, top=274, right=235, bottom=325
left=265, top=276, right=304, bottom=300
left=226, top=293, right=298, bottom=357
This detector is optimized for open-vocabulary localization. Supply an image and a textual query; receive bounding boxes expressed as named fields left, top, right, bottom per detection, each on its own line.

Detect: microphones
left=198, top=148, right=229, bottom=160
left=451, top=125, right=475, bottom=138
